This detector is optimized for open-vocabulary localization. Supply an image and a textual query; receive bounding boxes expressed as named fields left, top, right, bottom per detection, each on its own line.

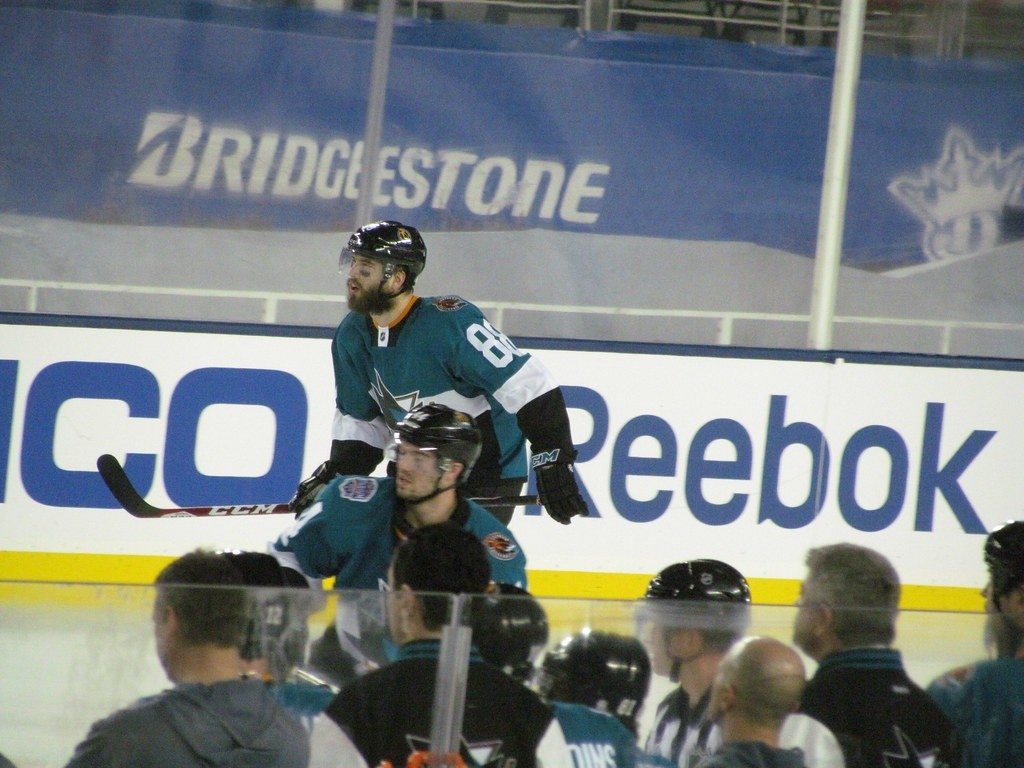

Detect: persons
left=65, top=521, right=1023, bottom=768
left=290, top=222, right=591, bottom=526
left=264, top=406, right=527, bottom=693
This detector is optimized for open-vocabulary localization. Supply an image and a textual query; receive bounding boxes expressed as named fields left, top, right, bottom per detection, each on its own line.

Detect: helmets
left=644, top=559, right=752, bottom=626
left=983, top=521, right=1024, bottom=597
left=538, top=631, right=653, bottom=720
left=395, top=401, right=483, bottom=483
left=466, top=582, right=550, bottom=683
left=343, top=221, right=427, bottom=274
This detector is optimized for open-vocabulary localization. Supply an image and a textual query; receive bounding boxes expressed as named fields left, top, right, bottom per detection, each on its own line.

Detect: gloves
left=287, top=461, right=344, bottom=520
left=530, top=448, right=589, bottom=525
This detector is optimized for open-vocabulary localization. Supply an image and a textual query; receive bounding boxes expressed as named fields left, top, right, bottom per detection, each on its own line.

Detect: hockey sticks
left=92, top=450, right=549, bottom=522
